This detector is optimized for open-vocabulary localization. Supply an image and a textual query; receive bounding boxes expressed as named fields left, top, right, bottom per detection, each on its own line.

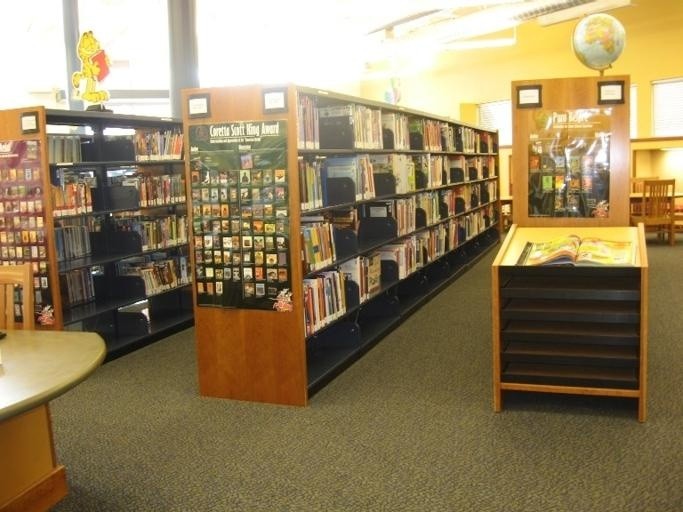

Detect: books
left=46, top=128, right=191, bottom=306
left=295, top=92, right=497, bottom=337
left=513, top=233, right=638, bottom=271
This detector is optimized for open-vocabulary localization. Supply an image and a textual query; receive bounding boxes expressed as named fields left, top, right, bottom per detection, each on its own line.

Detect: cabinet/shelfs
left=488, top=79, right=649, bottom=425
left=1, top=103, right=193, bottom=364
left=178, top=81, right=502, bottom=414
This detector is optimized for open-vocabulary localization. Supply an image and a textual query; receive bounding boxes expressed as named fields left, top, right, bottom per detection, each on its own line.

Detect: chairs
left=630, top=174, right=676, bottom=248
left=1, top=259, right=38, bottom=331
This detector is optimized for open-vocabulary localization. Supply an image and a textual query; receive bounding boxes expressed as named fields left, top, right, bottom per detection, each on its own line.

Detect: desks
left=0, top=324, right=109, bottom=512
left=627, top=189, right=683, bottom=241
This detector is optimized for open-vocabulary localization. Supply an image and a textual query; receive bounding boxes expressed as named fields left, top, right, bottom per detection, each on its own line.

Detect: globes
left=571, top=13, right=626, bottom=76
